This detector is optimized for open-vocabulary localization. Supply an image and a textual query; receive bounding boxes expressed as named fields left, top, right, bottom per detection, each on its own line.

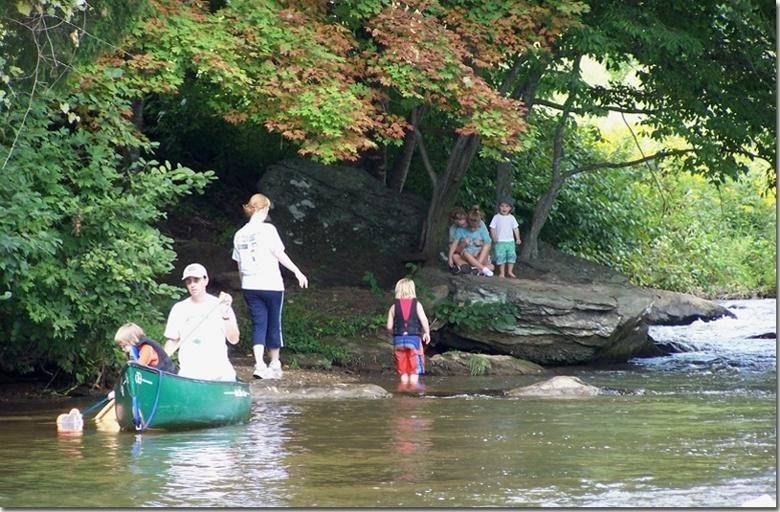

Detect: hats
left=180, top=263, right=208, bottom=280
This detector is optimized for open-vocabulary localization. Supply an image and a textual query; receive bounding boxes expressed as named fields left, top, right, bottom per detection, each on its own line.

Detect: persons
left=386, top=278, right=431, bottom=389
left=107, top=322, right=177, bottom=402
left=231, top=193, right=309, bottom=379
left=163, top=263, right=241, bottom=382
left=489, top=199, right=521, bottom=278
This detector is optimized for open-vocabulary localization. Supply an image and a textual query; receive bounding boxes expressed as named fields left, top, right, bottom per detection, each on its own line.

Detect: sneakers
left=269, top=360, right=283, bottom=379
left=449, top=264, right=495, bottom=277
left=398, top=382, right=424, bottom=390
left=252, top=363, right=269, bottom=379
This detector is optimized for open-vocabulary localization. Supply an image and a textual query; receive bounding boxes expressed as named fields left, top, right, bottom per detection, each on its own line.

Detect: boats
left=114, top=360, right=252, bottom=432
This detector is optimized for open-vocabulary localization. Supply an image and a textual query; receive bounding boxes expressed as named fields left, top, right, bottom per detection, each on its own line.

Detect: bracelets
left=223, top=317, right=231, bottom=320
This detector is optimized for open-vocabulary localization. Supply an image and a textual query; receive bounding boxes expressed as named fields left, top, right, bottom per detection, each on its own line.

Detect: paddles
left=95, top=301, right=223, bottom=420
